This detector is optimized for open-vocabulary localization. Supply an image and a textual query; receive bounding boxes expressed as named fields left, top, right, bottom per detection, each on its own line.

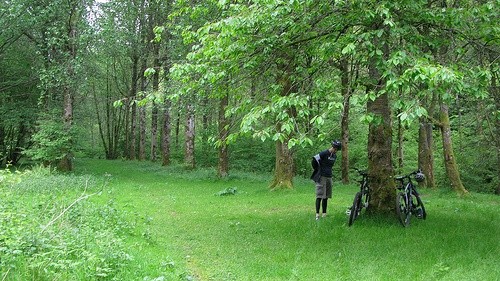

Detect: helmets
left=415, top=173, right=426, bottom=183
left=331, top=140, right=343, bottom=151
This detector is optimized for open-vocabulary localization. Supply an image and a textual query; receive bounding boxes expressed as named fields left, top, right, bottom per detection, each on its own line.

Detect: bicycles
left=345, top=166, right=376, bottom=227
left=393, top=167, right=427, bottom=227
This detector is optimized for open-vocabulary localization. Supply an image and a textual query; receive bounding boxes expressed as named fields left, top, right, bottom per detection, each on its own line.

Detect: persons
left=311, top=142, right=342, bottom=219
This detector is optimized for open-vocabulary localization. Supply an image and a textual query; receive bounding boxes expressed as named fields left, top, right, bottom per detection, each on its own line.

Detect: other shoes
left=315, top=213, right=319, bottom=220
left=322, top=213, right=326, bottom=217
